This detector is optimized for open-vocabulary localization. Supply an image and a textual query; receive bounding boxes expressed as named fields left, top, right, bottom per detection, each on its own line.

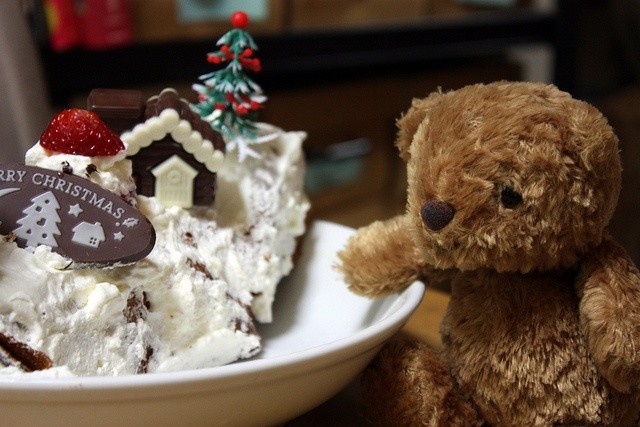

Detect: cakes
left=0, top=106, right=313, bottom=380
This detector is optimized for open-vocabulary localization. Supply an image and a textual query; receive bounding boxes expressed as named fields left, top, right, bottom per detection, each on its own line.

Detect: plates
left=1, top=216, right=427, bottom=425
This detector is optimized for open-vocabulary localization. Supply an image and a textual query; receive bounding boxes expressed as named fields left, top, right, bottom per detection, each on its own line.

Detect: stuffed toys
left=333, top=81, right=640, bottom=427
